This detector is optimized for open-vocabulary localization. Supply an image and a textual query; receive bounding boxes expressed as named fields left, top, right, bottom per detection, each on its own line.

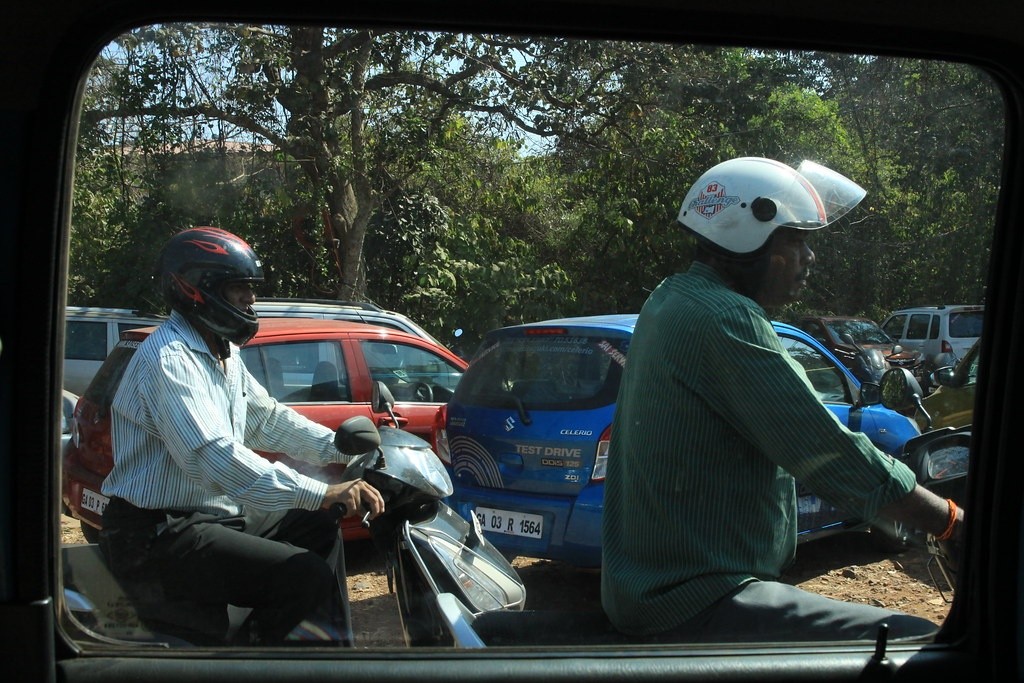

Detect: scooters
left=436, top=368, right=973, bottom=647
left=54, top=378, right=527, bottom=654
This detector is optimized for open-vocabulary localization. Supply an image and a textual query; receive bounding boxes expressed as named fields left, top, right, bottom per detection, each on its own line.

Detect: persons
left=603, top=157, right=965, bottom=643
left=100, top=228, right=385, bottom=646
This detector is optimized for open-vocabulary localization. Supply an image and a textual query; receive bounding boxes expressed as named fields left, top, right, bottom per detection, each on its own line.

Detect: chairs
left=310, top=358, right=339, bottom=402
left=261, top=355, right=290, bottom=401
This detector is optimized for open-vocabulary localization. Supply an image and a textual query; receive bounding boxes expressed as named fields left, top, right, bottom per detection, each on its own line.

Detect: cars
left=911, top=338, right=981, bottom=434
left=445, top=312, right=925, bottom=584
left=60, top=318, right=472, bottom=559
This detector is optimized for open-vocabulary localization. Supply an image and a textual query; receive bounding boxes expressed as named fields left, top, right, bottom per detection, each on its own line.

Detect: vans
left=880, top=306, right=989, bottom=376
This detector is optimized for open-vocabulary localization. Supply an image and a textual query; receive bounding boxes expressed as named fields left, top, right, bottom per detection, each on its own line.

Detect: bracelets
left=937, top=499, right=957, bottom=543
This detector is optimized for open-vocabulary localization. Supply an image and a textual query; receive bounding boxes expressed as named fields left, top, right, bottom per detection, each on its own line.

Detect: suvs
left=784, top=315, right=918, bottom=377
left=61, top=307, right=174, bottom=398
left=250, top=296, right=467, bottom=401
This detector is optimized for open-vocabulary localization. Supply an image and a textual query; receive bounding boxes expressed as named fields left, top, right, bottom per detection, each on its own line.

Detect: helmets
left=149, top=226, right=261, bottom=346
left=677, top=156, right=866, bottom=253
